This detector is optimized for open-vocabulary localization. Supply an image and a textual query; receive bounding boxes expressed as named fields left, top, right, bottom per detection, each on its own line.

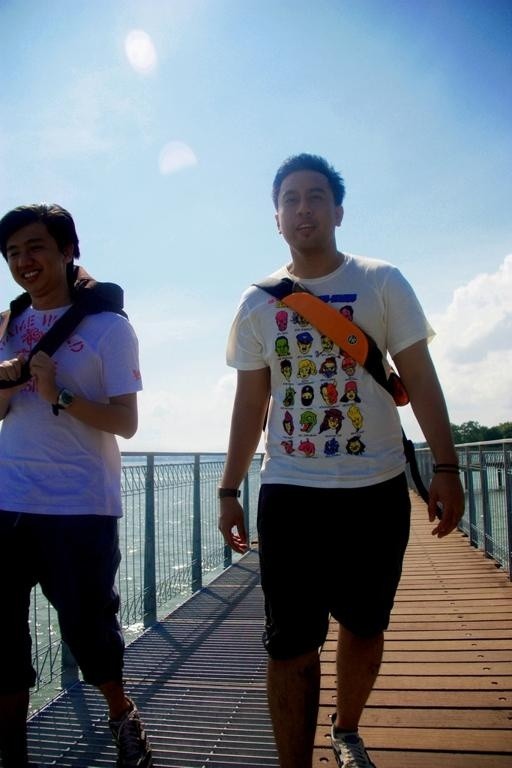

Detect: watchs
left=51, top=387, right=74, bottom=416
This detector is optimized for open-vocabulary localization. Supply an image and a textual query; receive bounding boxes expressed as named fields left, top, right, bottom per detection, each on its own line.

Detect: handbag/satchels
left=0, top=265, right=128, bottom=389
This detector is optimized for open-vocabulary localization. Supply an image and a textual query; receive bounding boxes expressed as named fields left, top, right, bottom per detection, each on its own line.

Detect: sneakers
left=108, top=695, right=152, bottom=768
left=0, top=692, right=28, bottom=768
left=331, top=712, right=375, bottom=768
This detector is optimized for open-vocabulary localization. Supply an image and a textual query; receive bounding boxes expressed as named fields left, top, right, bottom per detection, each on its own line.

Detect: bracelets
left=432, top=462, right=460, bottom=474
left=217, top=487, right=242, bottom=499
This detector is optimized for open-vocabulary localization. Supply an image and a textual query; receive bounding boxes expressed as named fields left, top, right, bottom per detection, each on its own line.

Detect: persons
left=215, top=151, right=465, bottom=768
left=0, top=202, right=157, bottom=767
